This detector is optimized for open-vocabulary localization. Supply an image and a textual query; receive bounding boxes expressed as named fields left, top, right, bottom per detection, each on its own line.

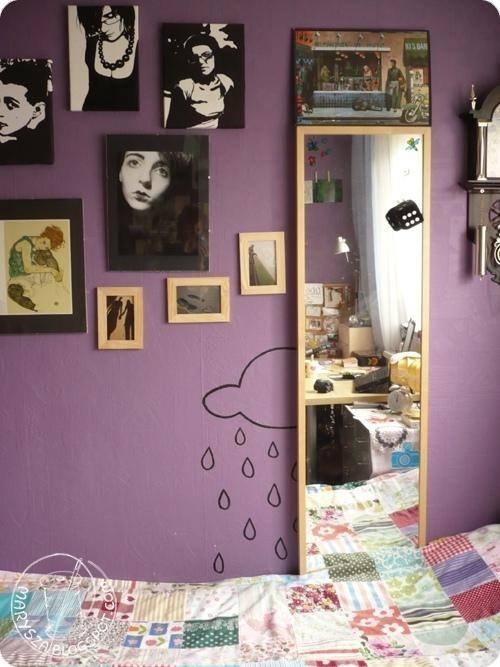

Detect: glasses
left=191, top=51, right=214, bottom=62
left=101, top=13, right=122, bottom=26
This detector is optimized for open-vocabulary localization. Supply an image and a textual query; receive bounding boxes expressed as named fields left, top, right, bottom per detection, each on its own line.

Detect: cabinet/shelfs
left=338, top=402, right=419, bottom=482
left=336, top=322, right=376, bottom=359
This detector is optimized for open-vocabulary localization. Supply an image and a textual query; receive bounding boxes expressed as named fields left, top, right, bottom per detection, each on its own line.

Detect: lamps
left=334, top=234, right=361, bottom=325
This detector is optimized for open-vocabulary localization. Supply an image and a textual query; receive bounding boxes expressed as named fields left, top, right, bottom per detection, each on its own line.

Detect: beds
left=306, top=467, right=420, bottom=572
left=0, top=521, right=500, bottom=667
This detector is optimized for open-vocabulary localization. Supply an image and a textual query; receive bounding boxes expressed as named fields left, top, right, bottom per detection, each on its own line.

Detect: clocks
left=385, top=382, right=414, bottom=412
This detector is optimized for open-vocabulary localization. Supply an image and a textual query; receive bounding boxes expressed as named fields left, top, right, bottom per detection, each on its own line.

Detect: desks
left=305, top=356, right=391, bottom=483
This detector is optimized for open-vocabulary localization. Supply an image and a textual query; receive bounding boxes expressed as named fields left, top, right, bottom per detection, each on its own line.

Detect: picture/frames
left=165, top=275, right=232, bottom=324
left=0, top=196, right=90, bottom=335
left=237, top=230, right=287, bottom=297
left=102, top=130, right=212, bottom=274
left=95, top=285, right=146, bottom=352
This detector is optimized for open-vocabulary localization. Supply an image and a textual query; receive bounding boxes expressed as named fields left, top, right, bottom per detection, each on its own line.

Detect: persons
left=119, top=299, right=135, bottom=340
left=6, top=222, right=67, bottom=316
left=75, top=4, right=136, bottom=110
left=321, top=58, right=339, bottom=85
left=361, top=60, right=375, bottom=87
left=163, top=32, right=241, bottom=129
left=0, top=59, right=49, bottom=164
left=107, top=296, right=124, bottom=338
left=297, top=55, right=318, bottom=114
left=384, top=56, right=406, bottom=114
left=116, top=149, right=197, bottom=254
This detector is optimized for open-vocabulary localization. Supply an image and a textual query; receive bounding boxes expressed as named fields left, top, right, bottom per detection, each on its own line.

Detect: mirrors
left=293, top=122, right=428, bottom=575
